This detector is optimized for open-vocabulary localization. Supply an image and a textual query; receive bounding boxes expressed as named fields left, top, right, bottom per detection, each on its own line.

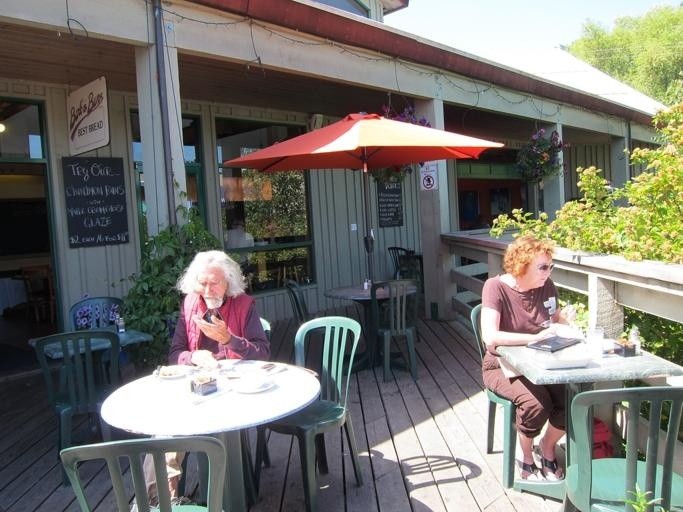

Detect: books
left=525, top=335, right=581, bottom=353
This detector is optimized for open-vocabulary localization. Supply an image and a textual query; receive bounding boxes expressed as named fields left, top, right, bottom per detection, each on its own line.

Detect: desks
left=28, top=326, right=154, bottom=393
left=324, top=281, right=417, bottom=375
left=101, top=358, right=321, bottom=512
left=496, top=338, right=682, bottom=503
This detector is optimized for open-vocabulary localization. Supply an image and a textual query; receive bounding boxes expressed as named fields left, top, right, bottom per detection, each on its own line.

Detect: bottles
left=363, top=279, right=368, bottom=289
left=118, top=318, right=125, bottom=333
left=367, top=280, right=372, bottom=297
left=115, top=315, right=120, bottom=333
left=629, top=325, right=640, bottom=355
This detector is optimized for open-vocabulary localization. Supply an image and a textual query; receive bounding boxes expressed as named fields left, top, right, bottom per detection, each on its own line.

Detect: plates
left=237, top=374, right=277, bottom=393
left=153, top=365, right=185, bottom=379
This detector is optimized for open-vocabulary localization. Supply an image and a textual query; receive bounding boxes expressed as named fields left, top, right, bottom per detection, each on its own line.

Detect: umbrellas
left=222, top=111, right=505, bottom=286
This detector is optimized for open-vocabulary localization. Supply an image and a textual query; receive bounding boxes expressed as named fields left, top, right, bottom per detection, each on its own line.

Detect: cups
left=585, top=326, right=604, bottom=366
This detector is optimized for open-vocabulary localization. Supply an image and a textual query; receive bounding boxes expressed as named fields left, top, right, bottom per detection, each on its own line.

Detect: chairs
left=387, top=247, right=417, bottom=281
left=19, top=264, right=49, bottom=323
left=69, top=296, right=123, bottom=334
left=36, top=331, right=120, bottom=486
left=60, top=437, right=227, bottom=512
left=254, top=316, right=363, bottom=510
left=370, top=279, right=418, bottom=383
left=284, top=280, right=367, bottom=376
left=562, top=386, right=683, bottom=512
left=471, top=304, right=517, bottom=488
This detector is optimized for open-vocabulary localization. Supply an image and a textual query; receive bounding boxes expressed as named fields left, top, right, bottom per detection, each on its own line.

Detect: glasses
left=529, top=263, right=555, bottom=273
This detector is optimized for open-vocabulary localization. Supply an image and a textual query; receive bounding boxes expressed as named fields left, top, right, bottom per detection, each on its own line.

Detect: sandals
left=537, top=437, right=565, bottom=481
left=515, top=454, right=543, bottom=482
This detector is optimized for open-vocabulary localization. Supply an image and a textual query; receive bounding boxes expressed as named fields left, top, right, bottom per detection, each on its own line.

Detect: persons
left=169, top=250, right=271, bottom=365
left=224, top=220, right=257, bottom=261
left=481, top=234, right=567, bottom=482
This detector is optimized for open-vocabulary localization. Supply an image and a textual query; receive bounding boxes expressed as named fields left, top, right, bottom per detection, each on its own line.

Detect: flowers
left=514, top=129, right=573, bottom=183
left=380, top=104, right=432, bottom=175
